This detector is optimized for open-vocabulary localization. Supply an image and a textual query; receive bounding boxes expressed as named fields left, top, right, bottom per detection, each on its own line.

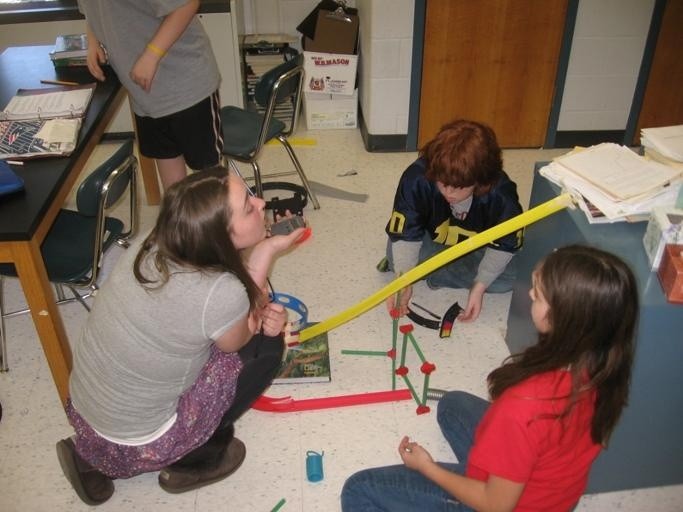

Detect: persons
left=341, top=245, right=640, bottom=512
left=377, top=120, right=524, bottom=322
left=77, top=0, right=222, bottom=193
left=56, top=165, right=312, bottom=506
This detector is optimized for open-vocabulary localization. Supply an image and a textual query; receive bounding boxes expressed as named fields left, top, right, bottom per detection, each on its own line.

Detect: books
left=0, top=82, right=98, bottom=160
left=242, top=34, right=295, bottom=131
left=270, top=321, right=331, bottom=384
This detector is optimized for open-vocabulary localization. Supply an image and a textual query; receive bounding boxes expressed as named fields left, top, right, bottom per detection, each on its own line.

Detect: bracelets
left=147, top=44, right=165, bottom=56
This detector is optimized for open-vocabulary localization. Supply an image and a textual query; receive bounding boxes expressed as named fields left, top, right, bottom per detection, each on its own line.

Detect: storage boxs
left=302, top=88, right=359, bottom=131
left=299, top=33, right=360, bottom=96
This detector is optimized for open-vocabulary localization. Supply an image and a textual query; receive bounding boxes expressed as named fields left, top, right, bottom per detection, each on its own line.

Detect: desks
left=0, top=43, right=162, bottom=425
left=504, top=161, right=683, bottom=494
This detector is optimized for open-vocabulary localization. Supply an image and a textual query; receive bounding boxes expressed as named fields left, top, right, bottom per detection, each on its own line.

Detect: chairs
left=0, top=136, right=140, bottom=374
left=219, top=53, right=320, bottom=211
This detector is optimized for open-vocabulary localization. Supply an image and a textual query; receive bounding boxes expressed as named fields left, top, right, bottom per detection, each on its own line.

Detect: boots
left=56, top=435, right=114, bottom=505
left=159, top=424, right=246, bottom=494
